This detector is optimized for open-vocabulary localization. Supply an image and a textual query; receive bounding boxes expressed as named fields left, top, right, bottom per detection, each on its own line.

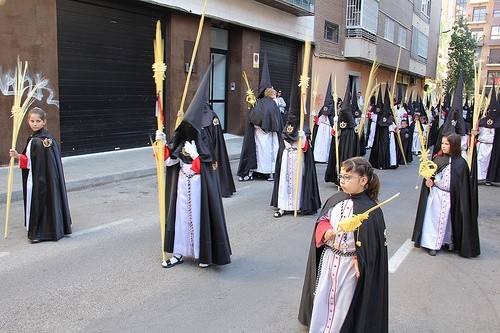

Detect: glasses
left=337, top=174, right=361, bottom=181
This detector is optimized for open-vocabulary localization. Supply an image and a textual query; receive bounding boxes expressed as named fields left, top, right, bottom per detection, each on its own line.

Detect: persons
left=156, top=58, right=233, bottom=268
left=269, top=61, right=322, bottom=218
left=297, top=157, right=389, bottom=333
left=9, top=107, right=72, bottom=241
left=210, top=64, right=500, bottom=199
left=412, top=132, right=481, bottom=258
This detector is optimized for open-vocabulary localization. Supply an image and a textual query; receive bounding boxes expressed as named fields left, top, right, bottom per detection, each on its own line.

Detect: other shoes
left=429, top=250, right=437, bottom=256
left=486, top=182, right=491, bottom=186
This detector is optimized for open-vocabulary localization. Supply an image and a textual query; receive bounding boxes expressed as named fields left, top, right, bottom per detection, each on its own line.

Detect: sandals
left=238, top=176, right=254, bottom=181
left=267, top=177, right=274, bottom=181
left=198, top=263, right=210, bottom=268
left=274, top=211, right=285, bottom=217
left=161, top=256, right=183, bottom=268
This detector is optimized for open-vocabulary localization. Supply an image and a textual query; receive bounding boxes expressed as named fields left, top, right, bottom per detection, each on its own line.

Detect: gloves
left=185, top=140, right=199, bottom=160
left=155, top=130, right=167, bottom=145
left=333, top=116, right=338, bottom=122
left=298, top=130, right=305, bottom=137
